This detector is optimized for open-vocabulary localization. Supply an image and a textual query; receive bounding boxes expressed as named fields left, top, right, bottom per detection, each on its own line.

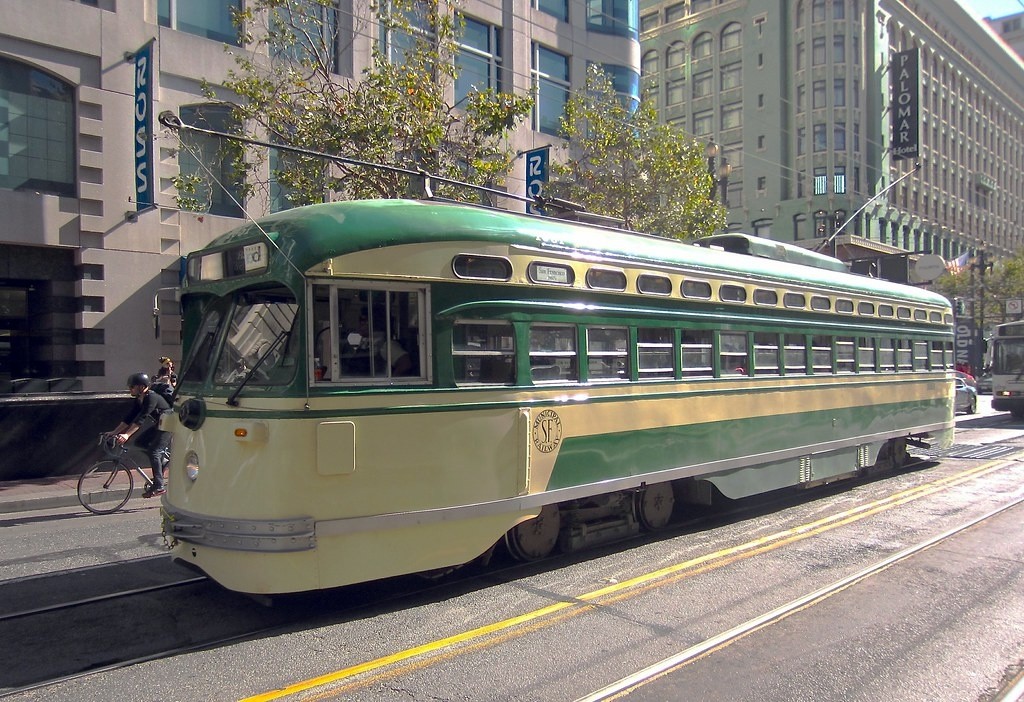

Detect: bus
left=982, top=320, right=1024, bottom=417
left=152, top=110, right=955, bottom=596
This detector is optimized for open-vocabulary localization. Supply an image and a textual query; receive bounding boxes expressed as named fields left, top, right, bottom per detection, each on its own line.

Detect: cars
left=955, top=371, right=977, bottom=388
left=955, top=377, right=978, bottom=415
left=976, top=370, right=993, bottom=395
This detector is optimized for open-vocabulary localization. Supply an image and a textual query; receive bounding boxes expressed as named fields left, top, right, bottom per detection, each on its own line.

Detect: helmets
left=127, top=373, right=149, bottom=386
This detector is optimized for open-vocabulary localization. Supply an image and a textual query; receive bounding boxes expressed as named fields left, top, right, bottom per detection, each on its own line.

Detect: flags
left=948, top=249, right=979, bottom=289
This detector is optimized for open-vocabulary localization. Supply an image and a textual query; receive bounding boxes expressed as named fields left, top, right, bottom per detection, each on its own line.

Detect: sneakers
left=143, top=487, right=166, bottom=498
left=162, top=458, right=170, bottom=479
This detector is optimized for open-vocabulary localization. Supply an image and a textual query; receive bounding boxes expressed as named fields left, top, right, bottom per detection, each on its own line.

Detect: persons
left=150, top=355, right=178, bottom=408
left=956, top=360, right=971, bottom=374
left=985, top=364, right=991, bottom=372
left=224, top=358, right=249, bottom=385
left=347, top=302, right=411, bottom=377
left=105, top=372, right=174, bottom=498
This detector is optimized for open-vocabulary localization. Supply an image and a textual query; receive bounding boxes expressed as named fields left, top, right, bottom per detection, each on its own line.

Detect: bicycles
left=76, top=432, right=171, bottom=515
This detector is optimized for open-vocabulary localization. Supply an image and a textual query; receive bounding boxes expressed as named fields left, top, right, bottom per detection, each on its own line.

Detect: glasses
left=129, top=385, right=135, bottom=389
